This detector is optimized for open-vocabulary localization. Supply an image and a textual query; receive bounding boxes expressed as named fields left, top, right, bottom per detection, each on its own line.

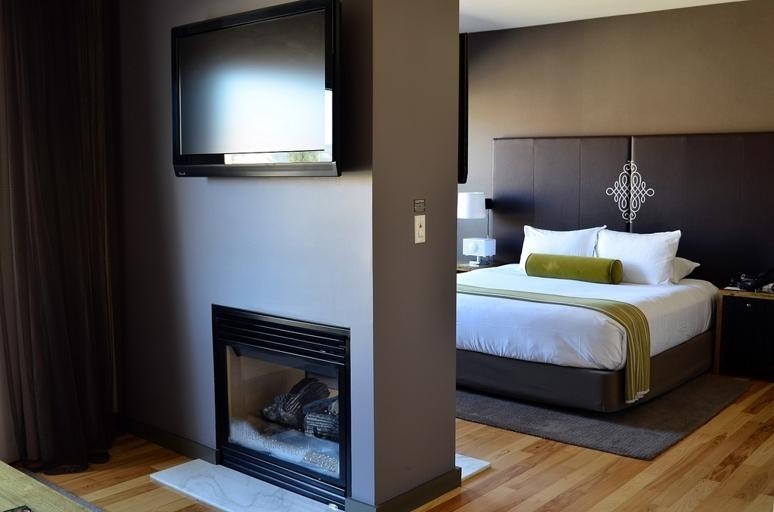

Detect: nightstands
left=457, top=264, right=490, bottom=273
left=713, top=289, right=774, bottom=384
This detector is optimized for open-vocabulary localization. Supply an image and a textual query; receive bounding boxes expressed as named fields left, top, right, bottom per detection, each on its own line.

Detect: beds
left=455, top=263, right=721, bottom=423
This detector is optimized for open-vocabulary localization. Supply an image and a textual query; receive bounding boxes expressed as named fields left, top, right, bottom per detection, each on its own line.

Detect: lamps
left=457, top=192, right=493, bottom=219
left=463, top=238, right=497, bottom=268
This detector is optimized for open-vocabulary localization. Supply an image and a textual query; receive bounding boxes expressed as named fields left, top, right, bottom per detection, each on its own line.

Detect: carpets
left=454, top=372, right=753, bottom=462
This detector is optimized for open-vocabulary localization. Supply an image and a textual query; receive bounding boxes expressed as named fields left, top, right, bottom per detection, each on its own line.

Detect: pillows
left=519, top=225, right=608, bottom=272
left=525, top=253, right=623, bottom=284
left=670, top=257, right=702, bottom=285
left=594, top=230, right=682, bottom=288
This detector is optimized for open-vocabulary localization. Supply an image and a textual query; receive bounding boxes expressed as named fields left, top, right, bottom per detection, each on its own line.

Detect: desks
left=0, top=456, right=107, bottom=512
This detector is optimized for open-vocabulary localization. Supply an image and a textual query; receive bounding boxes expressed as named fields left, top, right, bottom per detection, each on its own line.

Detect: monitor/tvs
left=458, top=32, right=469, bottom=184
left=171, top=0, right=344, bottom=179
